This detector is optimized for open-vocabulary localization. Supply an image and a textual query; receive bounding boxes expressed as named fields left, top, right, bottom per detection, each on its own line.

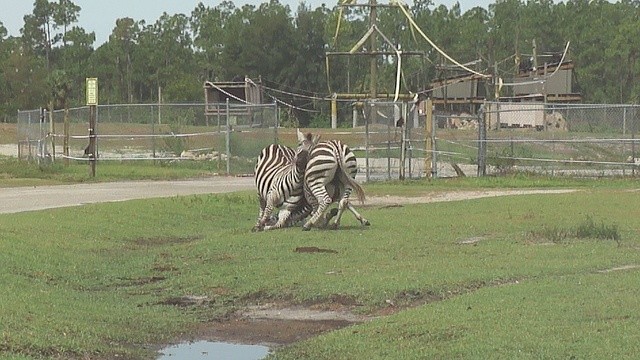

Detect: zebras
left=265, top=139, right=370, bottom=231
left=251, top=128, right=321, bottom=232
